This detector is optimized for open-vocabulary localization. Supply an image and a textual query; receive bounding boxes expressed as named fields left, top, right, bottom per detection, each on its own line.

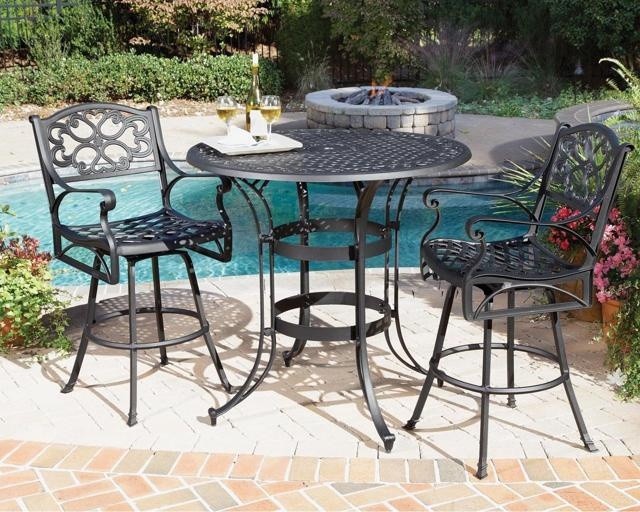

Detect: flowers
left=1, top=210, right=69, bottom=351
left=548, top=203, right=588, bottom=250
left=587, top=206, right=637, bottom=305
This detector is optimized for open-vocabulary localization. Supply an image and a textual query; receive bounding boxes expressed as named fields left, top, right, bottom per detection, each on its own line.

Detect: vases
left=597, top=294, right=637, bottom=356
left=2, top=308, right=28, bottom=350
left=568, top=262, right=608, bottom=323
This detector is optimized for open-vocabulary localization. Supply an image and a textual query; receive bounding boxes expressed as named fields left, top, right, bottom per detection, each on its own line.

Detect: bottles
left=245, top=53, right=263, bottom=132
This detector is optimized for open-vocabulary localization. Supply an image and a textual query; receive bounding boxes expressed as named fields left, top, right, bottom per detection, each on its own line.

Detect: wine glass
left=259, top=95, right=283, bottom=143
left=215, top=95, right=238, bottom=145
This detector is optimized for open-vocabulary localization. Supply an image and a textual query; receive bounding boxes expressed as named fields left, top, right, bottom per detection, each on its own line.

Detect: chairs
left=31, top=104, right=245, bottom=426
left=405, top=122, right=630, bottom=483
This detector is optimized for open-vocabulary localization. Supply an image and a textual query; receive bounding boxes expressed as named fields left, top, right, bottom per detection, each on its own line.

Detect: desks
left=187, top=125, right=472, bottom=452
